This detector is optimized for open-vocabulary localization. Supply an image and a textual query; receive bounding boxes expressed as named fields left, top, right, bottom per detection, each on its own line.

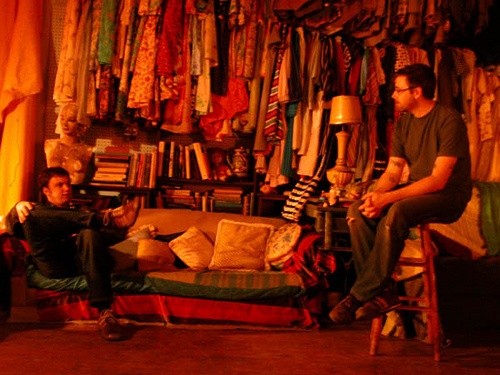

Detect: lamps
left=326, top=96, right=363, bottom=206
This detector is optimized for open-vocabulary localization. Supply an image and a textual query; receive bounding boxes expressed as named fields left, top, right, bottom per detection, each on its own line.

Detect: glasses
left=392, top=87, right=410, bottom=94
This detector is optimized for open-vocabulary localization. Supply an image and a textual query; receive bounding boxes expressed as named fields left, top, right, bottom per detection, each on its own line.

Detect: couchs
left=0, top=208, right=323, bottom=332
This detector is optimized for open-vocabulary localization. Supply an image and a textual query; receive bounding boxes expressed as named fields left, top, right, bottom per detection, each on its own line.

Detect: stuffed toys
left=207, top=151, right=232, bottom=181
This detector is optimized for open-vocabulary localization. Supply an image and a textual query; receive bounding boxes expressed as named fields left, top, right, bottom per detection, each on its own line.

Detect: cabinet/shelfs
left=85, top=181, right=355, bottom=252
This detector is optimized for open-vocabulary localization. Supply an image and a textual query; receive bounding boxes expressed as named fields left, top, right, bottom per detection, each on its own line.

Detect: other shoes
left=102, top=196, right=141, bottom=234
left=97, top=309, right=127, bottom=340
left=328, top=289, right=402, bottom=323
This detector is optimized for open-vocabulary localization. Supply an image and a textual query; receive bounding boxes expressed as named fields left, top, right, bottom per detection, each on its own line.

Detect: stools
left=369, top=224, right=441, bottom=360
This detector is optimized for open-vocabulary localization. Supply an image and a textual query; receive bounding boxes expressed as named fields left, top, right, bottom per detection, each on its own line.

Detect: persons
left=45, top=103, right=93, bottom=183
left=5, top=166, right=139, bottom=341
left=329, top=63, right=471, bottom=322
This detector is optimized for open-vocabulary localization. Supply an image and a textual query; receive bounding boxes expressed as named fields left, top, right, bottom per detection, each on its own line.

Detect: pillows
left=111, top=218, right=302, bottom=274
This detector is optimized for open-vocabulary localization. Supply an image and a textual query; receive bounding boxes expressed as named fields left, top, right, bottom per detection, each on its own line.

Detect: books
left=79, top=185, right=254, bottom=216
left=93, top=137, right=211, bottom=189
